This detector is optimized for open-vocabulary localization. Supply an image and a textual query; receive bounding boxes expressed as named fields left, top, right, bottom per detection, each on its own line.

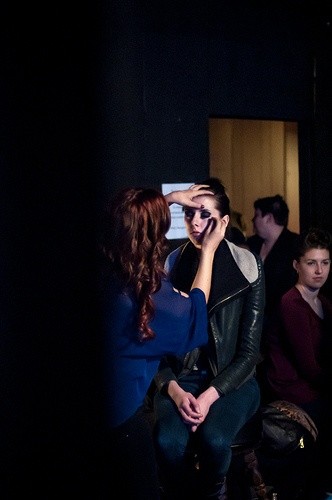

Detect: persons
left=101, top=179, right=230, bottom=497
left=247, top=194, right=305, bottom=372
left=265, top=231, right=332, bottom=499
left=146, top=178, right=266, bottom=500
